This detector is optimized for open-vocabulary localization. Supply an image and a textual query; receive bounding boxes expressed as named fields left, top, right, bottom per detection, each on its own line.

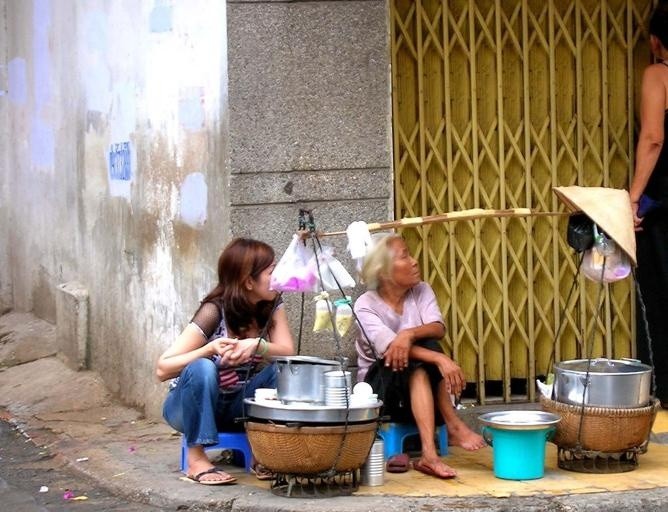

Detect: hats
left=551, top=186, right=640, bottom=269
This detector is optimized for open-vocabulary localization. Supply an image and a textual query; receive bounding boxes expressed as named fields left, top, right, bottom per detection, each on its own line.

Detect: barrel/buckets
left=272, top=357, right=343, bottom=404
left=481, top=426, right=559, bottom=484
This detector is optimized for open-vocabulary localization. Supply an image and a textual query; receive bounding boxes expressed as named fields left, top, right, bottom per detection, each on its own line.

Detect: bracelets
left=256, top=338, right=268, bottom=356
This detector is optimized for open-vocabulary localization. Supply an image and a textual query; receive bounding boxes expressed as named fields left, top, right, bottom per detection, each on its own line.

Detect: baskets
left=539, top=392, right=660, bottom=452
left=243, top=421, right=379, bottom=474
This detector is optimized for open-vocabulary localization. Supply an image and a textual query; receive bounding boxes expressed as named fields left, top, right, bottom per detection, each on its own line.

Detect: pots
left=268, top=352, right=352, bottom=404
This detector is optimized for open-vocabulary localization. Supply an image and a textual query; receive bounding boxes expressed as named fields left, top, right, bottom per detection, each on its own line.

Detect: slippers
left=413, top=459, right=457, bottom=479
left=249, top=463, right=277, bottom=480
left=179, top=467, right=237, bottom=484
left=386, top=454, right=408, bottom=471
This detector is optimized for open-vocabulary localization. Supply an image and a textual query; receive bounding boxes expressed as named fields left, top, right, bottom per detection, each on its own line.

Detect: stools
left=182, top=434, right=251, bottom=474
left=374, top=419, right=449, bottom=463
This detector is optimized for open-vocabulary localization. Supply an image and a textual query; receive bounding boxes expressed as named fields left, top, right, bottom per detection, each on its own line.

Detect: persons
left=353, top=234, right=488, bottom=478
left=630, top=4, right=668, bottom=410
left=156, top=238, right=294, bottom=485
